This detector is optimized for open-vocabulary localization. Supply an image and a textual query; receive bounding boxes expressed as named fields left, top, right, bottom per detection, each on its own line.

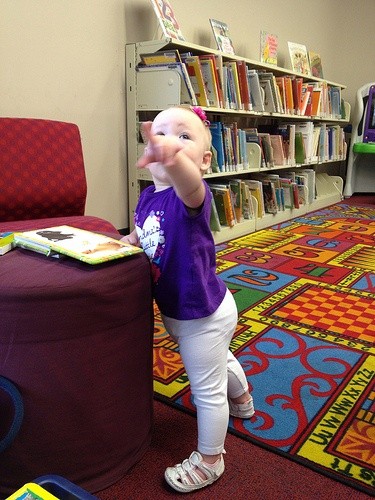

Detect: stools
left=0, top=231, right=152, bottom=495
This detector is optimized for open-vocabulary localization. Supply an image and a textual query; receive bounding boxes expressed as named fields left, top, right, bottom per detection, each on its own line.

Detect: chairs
left=344, top=82, right=375, bottom=199
left=0, top=117, right=121, bottom=241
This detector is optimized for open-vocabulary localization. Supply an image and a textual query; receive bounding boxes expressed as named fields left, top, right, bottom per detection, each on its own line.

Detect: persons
left=119, top=107, right=256, bottom=493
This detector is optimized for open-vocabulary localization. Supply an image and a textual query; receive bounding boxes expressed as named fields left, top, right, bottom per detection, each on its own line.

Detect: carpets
left=153, top=196, right=375, bottom=499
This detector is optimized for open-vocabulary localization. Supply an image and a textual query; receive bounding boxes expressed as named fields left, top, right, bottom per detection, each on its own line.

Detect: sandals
left=165, top=452, right=225, bottom=492
left=228, top=396, right=256, bottom=418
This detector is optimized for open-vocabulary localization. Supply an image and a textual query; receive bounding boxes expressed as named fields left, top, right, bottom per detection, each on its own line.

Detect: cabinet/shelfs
left=126, top=38, right=350, bottom=246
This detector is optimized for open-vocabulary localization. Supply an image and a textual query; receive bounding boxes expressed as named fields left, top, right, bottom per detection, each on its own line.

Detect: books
left=140, top=49, right=347, bottom=232
left=308, top=51, right=323, bottom=78
left=260, top=31, right=279, bottom=66
left=14, top=225, right=143, bottom=265
left=209, top=19, right=236, bottom=55
left=152, top=0, right=184, bottom=40
left=288, top=42, right=311, bottom=75
